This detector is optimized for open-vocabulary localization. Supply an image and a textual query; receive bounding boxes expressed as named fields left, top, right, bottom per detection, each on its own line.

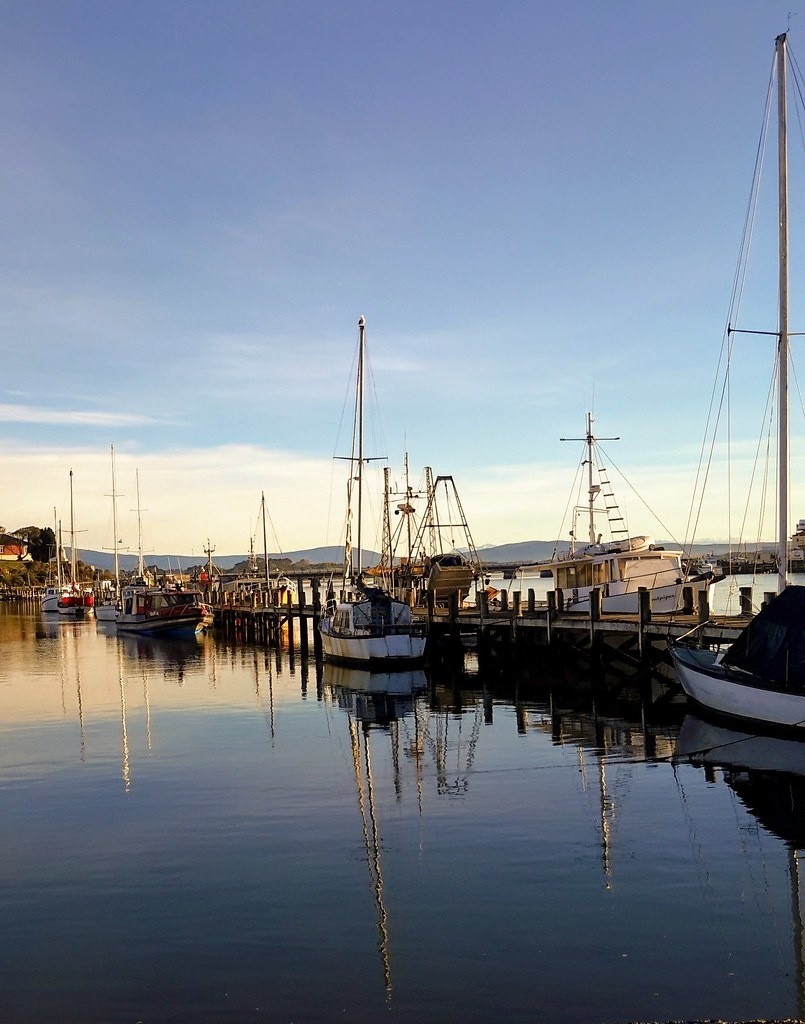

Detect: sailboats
left=316, top=315, right=429, bottom=664
left=669, top=712, right=804, bottom=1024
left=320, top=662, right=429, bottom=1014
left=664, top=29, right=805, bottom=730
left=35, top=312, right=295, bottom=634
left=463, top=412, right=725, bottom=614
